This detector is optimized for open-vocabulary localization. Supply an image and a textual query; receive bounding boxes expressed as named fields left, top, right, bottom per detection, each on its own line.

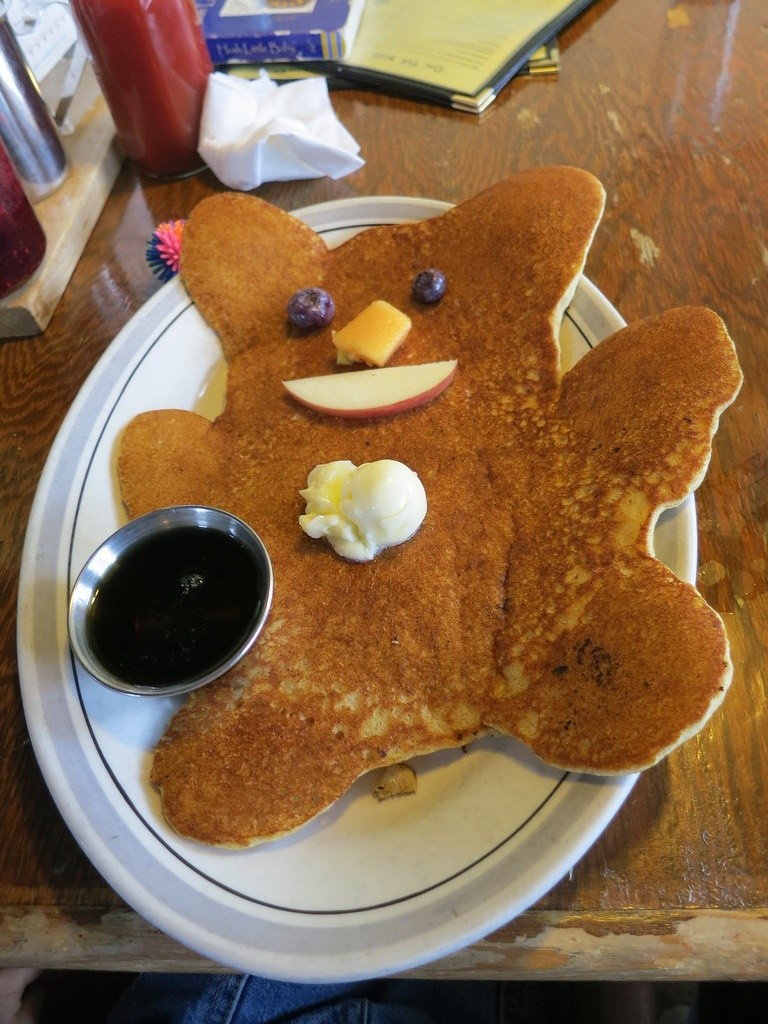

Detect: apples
left=280, top=359, right=459, bottom=419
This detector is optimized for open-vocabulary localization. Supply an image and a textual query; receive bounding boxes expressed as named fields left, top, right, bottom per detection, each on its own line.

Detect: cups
left=0, top=12, right=71, bottom=199
left=68, top=2, right=220, bottom=180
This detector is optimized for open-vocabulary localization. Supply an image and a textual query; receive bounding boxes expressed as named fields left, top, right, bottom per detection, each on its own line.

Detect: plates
left=69, top=504, right=274, bottom=697
left=16, top=194, right=699, bottom=985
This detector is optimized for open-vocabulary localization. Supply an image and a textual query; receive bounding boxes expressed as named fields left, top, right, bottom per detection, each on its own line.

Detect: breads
left=116, top=164, right=742, bottom=846
